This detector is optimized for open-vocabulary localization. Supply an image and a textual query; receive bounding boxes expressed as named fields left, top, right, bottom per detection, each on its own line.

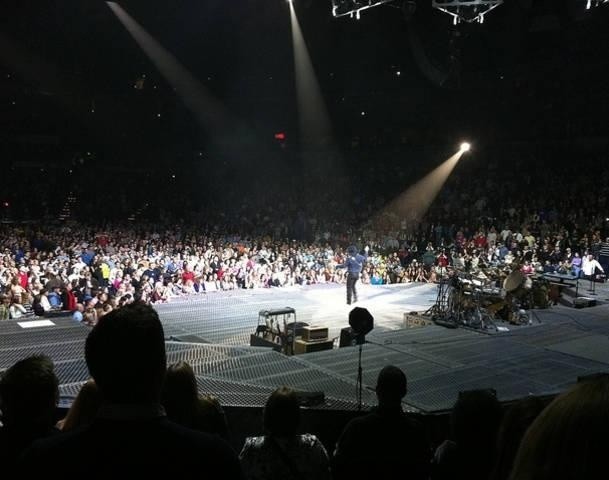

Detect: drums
left=504, top=272, right=532, bottom=296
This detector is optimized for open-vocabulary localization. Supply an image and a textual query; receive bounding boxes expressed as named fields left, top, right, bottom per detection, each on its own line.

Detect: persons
left=1, top=161, right=609, bottom=325
left=22, top=300, right=246, bottom=480
left=1, top=354, right=609, bottom=480
